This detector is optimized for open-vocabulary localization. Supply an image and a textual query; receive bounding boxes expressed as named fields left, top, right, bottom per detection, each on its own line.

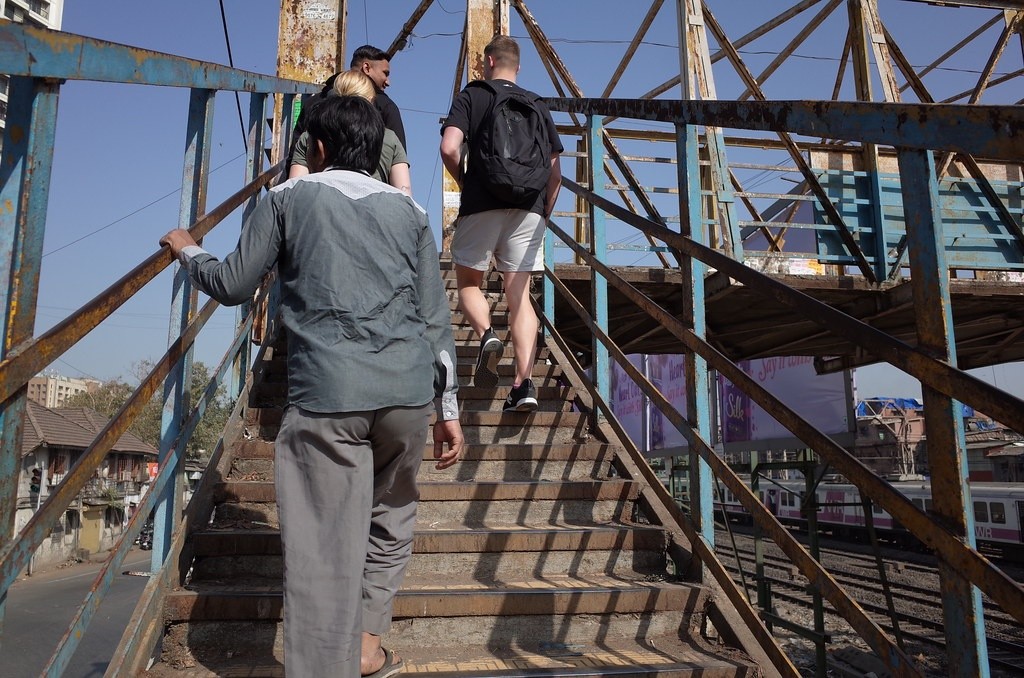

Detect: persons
left=437, top=34, right=564, bottom=411
left=285, top=41, right=407, bottom=170
left=158, top=94, right=460, bottom=678
left=288, top=67, right=411, bottom=199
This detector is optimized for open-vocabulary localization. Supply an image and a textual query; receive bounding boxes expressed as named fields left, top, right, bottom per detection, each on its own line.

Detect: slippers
left=362, top=645, right=408, bottom=678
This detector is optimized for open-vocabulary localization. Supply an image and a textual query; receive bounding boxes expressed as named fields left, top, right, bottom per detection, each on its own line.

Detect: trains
left=658, top=477, right=1022, bottom=555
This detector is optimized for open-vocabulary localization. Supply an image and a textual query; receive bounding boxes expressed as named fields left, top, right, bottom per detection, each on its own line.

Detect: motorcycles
left=139, top=526, right=154, bottom=551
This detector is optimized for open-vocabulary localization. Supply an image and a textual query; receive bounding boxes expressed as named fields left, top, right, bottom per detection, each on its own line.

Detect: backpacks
left=466, top=79, right=554, bottom=192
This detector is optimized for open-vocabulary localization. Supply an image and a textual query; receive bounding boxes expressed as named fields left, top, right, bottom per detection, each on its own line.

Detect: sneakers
left=503, top=381, right=538, bottom=412
left=472, top=331, right=505, bottom=388
